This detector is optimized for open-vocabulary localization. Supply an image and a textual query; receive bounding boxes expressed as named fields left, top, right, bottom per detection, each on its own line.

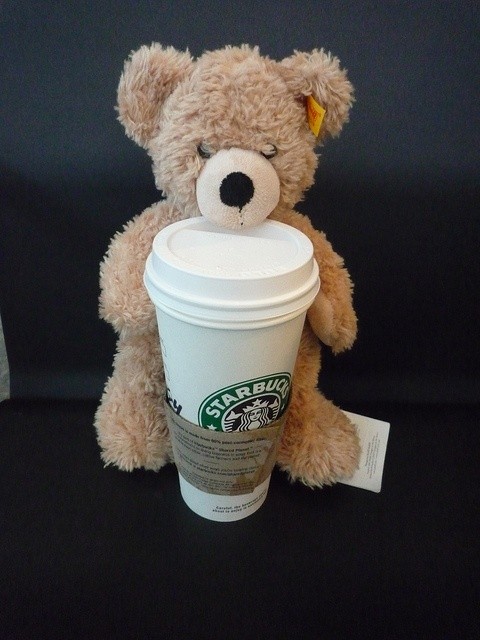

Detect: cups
left=146, top=216, right=322, bottom=523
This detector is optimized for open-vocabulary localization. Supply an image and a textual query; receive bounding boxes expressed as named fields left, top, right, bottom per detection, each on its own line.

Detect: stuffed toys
left=93, top=42, right=361, bottom=491
